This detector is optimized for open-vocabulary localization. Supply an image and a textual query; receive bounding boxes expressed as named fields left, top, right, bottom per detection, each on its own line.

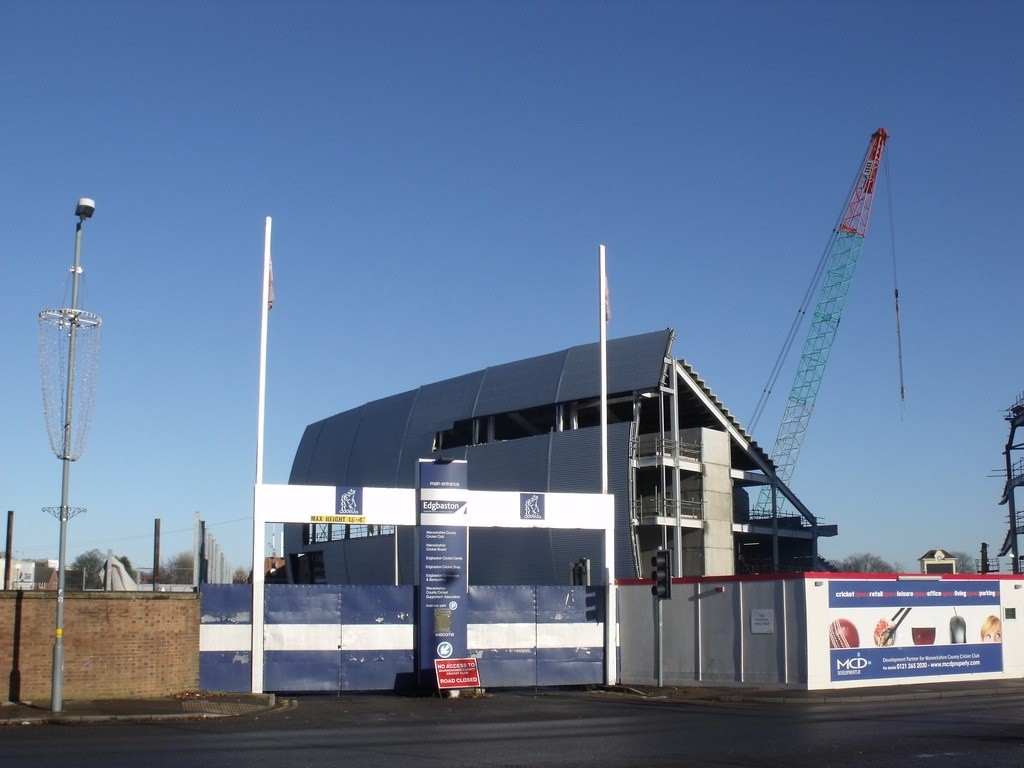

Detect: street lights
left=43, top=195, right=97, bottom=713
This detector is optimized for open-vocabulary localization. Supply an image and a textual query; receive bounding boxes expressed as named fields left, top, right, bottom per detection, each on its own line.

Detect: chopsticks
left=883, top=608, right=911, bottom=645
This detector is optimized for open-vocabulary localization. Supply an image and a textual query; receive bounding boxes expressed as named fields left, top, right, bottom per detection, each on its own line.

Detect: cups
left=912, top=628, right=935, bottom=645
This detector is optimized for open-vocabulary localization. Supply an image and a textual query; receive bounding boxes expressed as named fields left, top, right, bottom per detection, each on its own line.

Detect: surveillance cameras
left=73, top=198, right=94, bottom=218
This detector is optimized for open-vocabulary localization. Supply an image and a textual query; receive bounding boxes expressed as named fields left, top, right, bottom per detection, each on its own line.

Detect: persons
left=980, top=614, right=1002, bottom=643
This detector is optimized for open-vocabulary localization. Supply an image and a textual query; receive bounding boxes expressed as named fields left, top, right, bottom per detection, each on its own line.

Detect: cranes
left=752, top=125, right=892, bottom=516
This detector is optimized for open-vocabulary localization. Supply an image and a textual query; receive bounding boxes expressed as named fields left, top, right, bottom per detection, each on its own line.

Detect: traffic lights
left=650, top=549, right=673, bottom=604
